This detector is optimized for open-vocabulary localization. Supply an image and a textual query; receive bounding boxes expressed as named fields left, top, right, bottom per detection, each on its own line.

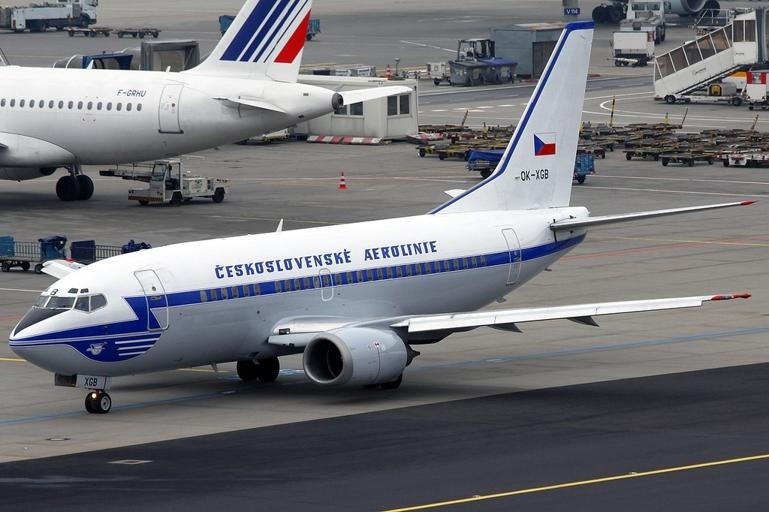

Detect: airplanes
left=0, top=0, right=413, bottom=202
left=9, top=20, right=760, bottom=417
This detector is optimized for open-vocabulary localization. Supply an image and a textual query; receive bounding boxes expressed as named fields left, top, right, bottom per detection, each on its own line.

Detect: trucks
left=128, top=158, right=233, bottom=207
left=619, top=0, right=671, bottom=45
left=0, top=0, right=98, bottom=33
